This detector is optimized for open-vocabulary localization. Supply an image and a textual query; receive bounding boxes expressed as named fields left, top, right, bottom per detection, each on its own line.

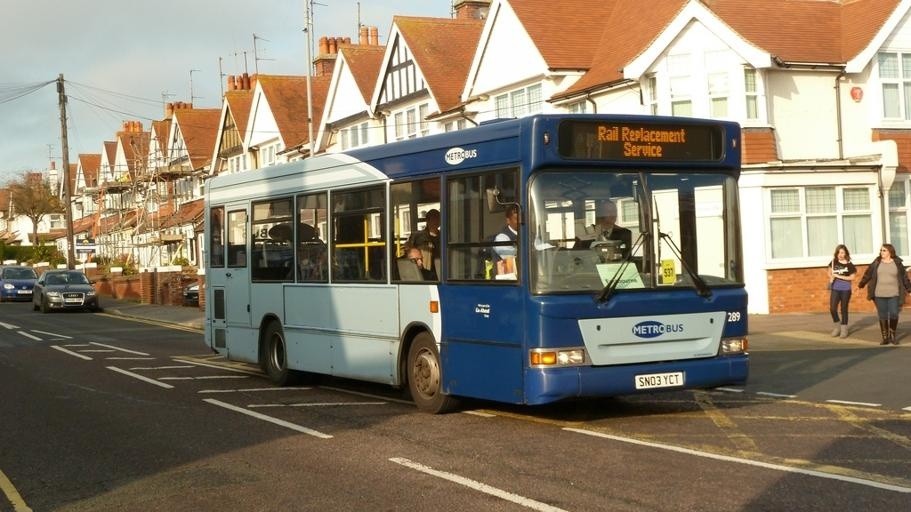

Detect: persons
left=826, top=243, right=858, bottom=339
left=402, top=208, right=441, bottom=280
left=490, top=206, right=520, bottom=279
left=570, top=198, right=632, bottom=261
left=854, top=243, right=911, bottom=345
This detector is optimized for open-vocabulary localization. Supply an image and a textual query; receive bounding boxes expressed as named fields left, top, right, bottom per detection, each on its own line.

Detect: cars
left=0, top=266, right=98, bottom=313
left=183, top=281, right=199, bottom=304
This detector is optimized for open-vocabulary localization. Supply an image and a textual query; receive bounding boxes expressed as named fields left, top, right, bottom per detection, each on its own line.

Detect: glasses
left=880, top=250, right=888, bottom=252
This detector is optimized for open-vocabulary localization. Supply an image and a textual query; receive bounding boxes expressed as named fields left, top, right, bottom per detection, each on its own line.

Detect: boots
left=839, top=324, right=849, bottom=339
left=831, top=321, right=842, bottom=338
left=879, top=319, right=889, bottom=346
left=888, top=317, right=900, bottom=346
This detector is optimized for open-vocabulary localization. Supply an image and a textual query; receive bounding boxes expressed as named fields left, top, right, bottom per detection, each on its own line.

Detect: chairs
left=395, top=257, right=425, bottom=281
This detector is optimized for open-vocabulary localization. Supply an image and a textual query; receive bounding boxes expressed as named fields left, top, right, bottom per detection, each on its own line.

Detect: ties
left=603, top=231, right=609, bottom=238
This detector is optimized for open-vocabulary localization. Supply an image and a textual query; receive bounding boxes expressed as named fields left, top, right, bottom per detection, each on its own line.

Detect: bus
left=204, top=114, right=749, bottom=414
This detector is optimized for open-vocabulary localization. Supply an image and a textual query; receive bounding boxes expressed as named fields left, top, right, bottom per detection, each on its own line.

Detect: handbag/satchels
left=827, top=277, right=836, bottom=290
